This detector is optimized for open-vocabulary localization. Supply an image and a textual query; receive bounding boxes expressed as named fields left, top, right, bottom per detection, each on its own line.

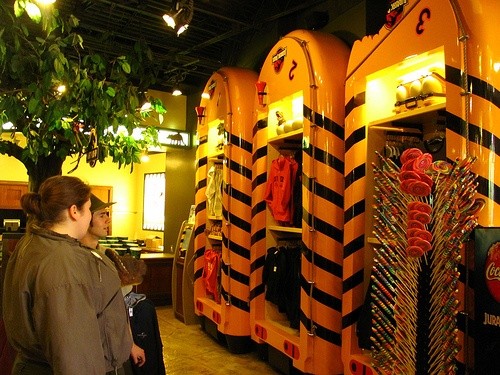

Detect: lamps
left=170, top=77, right=181, bottom=96
left=161, top=0, right=192, bottom=36
left=201, top=89, right=213, bottom=99
left=140, top=92, right=152, bottom=110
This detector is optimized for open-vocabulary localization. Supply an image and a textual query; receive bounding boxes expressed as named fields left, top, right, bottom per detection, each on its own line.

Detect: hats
left=90, top=191, right=117, bottom=214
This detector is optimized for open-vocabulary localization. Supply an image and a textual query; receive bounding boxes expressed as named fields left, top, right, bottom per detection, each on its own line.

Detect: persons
left=80, top=194, right=146, bottom=367
left=3, top=176, right=132, bottom=375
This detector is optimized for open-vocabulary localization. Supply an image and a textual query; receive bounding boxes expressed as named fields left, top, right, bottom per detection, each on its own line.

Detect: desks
left=119, top=252, right=173, bottom=303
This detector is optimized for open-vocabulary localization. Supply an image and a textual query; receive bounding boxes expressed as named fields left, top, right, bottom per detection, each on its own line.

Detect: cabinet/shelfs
left=263, top=91, right=304, bottom=334
left=203, top=114, right=226, bottom=306
left=360, top=47, right=447, bottom=358
